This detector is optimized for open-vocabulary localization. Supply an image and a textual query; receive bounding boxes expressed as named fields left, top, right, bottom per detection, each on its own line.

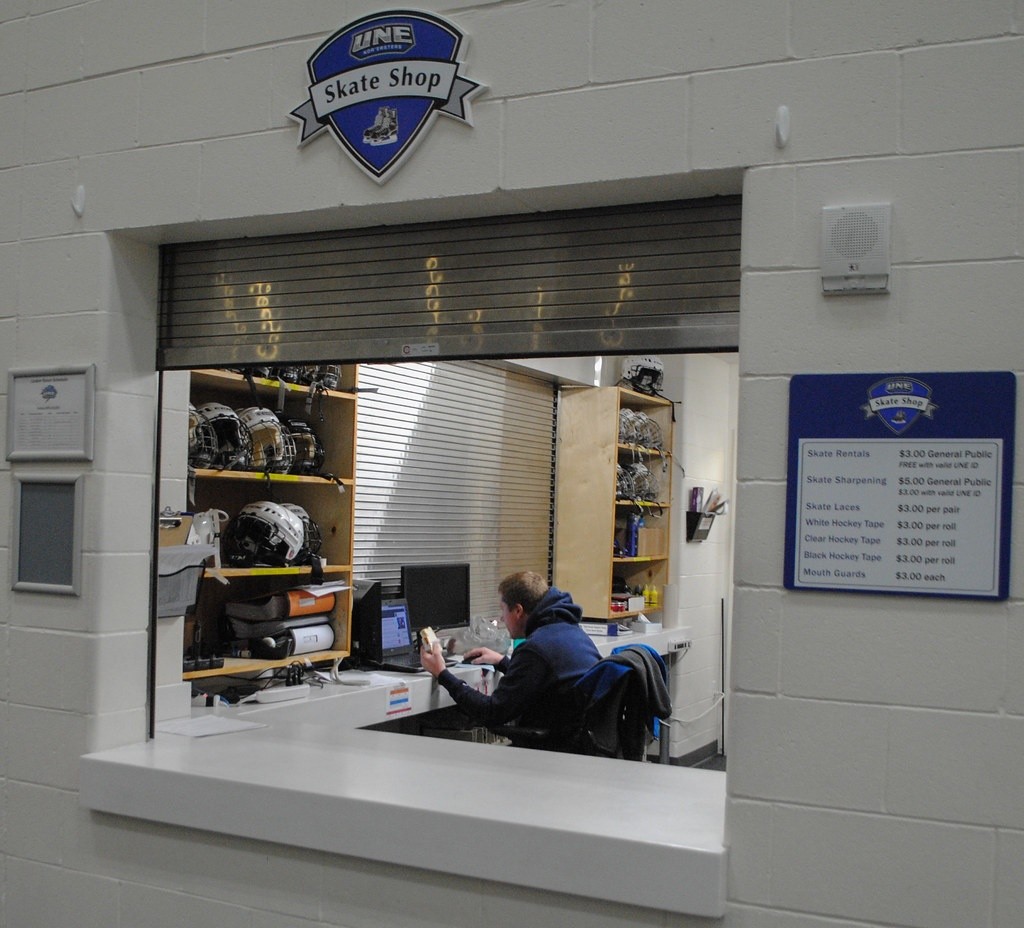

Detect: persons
left=420, top=571, right=605, bottom=752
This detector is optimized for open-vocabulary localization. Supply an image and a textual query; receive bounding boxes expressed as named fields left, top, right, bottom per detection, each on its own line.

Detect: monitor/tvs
left=350, top=579, right=382, bottom=672
left=401, top=562, right=471, bottom=653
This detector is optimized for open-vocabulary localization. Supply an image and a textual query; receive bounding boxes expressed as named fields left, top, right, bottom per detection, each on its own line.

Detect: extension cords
left=256, top=683, right=310, bottom=704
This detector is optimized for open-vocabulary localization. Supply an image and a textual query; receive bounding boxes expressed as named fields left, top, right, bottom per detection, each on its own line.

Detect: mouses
left=462, top=655, right=486, bottom=666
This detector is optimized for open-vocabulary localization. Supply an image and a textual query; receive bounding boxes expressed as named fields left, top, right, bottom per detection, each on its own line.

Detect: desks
left=222, top=626, right=691, bottom=767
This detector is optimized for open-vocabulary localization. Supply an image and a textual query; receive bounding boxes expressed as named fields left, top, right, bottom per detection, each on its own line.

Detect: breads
left=420, top=626, right=442, bottom=654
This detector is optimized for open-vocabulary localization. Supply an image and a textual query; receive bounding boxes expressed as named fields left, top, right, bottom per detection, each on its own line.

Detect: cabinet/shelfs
left=553, top=385, right=672, bottom=620
left=182, top=363, right=358, bottom=682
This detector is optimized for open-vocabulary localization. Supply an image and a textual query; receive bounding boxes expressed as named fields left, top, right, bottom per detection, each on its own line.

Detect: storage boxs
left=638, top=528, right=666, bottom=556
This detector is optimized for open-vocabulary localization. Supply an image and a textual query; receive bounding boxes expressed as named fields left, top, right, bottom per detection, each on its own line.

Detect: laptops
left=379, top=598, right=459, bottom=673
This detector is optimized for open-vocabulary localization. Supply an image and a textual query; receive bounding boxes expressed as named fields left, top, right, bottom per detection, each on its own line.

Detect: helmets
left=616, top=356, right=665, bottom=500
left=223, top=501, right=321, bottom=566
left=188, top=401, right=324, bottom=474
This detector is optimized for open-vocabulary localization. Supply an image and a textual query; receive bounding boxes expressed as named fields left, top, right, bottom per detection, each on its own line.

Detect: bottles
left=237, top=650, right=251, bottom=658
left=649, top=585, right=658, bottom=606
left=643, top=584, right=649, bottom=604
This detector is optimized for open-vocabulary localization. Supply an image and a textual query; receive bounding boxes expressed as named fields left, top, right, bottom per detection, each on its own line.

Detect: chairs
left=488, top=647, right=660, bottom=760
